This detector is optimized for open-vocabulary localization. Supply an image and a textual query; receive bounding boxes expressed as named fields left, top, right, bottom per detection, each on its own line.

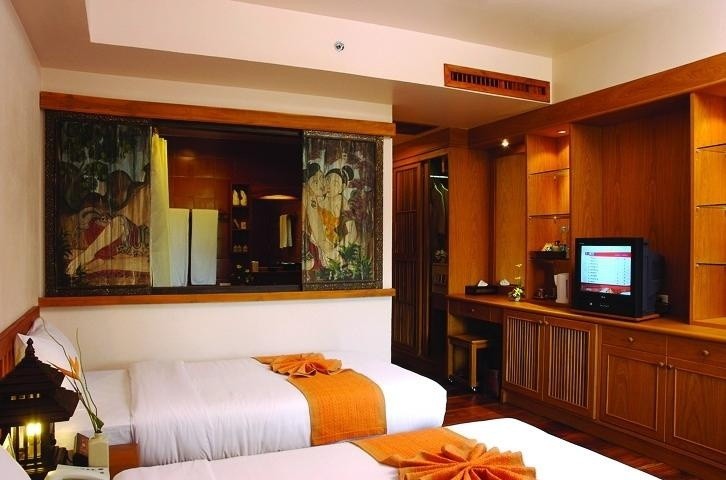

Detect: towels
left=280, top=214, right=293, bottom=249
left=169, top=207, right=219, bottom=287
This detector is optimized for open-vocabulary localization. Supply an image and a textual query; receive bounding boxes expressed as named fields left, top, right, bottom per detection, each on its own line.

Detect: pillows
left=14, top=316, right=80, bottom=394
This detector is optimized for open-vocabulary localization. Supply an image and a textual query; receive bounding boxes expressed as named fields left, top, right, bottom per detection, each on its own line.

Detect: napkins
left=476, top=280, right=489, bottom=288
left=46, top=464, right=112, bottom=480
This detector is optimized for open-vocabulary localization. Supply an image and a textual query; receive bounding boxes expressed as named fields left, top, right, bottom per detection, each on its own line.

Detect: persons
left=302, top=162, right=358, bottom=281
left=64, top=170, right=150, bottom=280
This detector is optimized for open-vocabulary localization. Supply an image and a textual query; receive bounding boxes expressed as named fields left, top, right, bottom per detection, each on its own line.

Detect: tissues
left=498, top=279, right=518, bottom=293
left=465, top=280, right=495, bottom=295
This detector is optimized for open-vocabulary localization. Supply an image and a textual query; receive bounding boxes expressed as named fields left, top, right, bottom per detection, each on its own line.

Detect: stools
left=449, top=333, right=500, bottom=395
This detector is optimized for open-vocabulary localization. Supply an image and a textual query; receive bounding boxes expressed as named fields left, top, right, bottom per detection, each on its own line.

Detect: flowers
left=508, top=263, right=523, bottom=298
left=44, top=328, right=104, bottom=434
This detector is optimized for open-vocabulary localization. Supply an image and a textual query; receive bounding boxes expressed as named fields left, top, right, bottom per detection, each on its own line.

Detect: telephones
left=44, top=464, right=111, bottom=480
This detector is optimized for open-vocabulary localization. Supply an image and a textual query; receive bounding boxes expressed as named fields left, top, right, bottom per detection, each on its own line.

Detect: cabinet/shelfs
left=502, top=309, right=599, bottom=420
left=397, top=130, right=467, bottom=387
left=465, top=51, right=722, bottom=328
left=595, top=326, right=722, bottom=464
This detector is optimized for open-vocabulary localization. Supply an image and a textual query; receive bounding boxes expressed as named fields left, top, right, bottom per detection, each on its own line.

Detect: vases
left=512, top=295, right=522, bottom=302
left=88, top=432, right=109, bottom=468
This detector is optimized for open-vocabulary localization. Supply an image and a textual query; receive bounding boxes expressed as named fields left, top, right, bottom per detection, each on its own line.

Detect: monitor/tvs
left=571, top=237, right=665, bottom=318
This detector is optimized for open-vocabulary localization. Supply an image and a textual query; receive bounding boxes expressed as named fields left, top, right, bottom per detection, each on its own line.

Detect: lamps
left=1, top=337, right=81, bottom=480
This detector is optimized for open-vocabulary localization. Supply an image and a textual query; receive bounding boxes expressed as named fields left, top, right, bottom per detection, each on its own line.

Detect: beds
left=0, top=307, right=447, bottom=472
left=0, top=418, right=663, bottom=480
